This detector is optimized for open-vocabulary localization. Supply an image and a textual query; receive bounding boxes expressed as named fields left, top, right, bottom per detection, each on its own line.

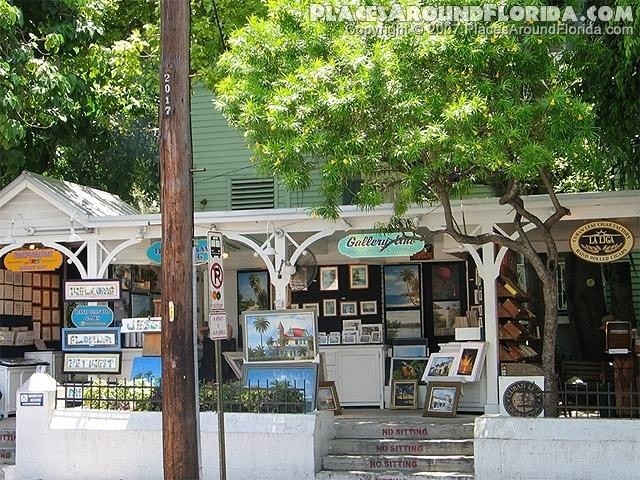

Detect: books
left=497, top=273, right=540, bottom=363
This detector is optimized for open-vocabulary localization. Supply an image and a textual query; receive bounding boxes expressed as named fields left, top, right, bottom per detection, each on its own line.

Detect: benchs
left=560, top=358, right=605, bottom=382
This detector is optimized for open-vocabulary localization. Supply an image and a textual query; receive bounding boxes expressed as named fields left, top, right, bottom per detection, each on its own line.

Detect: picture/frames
left=340, top=301, right=358, bottom=316
left=360, top=301, right=378, bottom=315
left=432, top=262, right=461, bottom=301
left=241, top=308, right=318, bottom=363
left=243, top=363, right=319, bottom=412
left=349, top=265, right=369, bottom=289
left=391, top=379, right=418, bottom=411
left=319, top=320, right=383, bottom=344
left=323, top=299, right=337, bottom=317
left=383, top=309, right=424, bottom=340
left=422, top=380, right=461, bottom=418
left=389, top=342, right=487, bottom=383
left=316, top=380, right=343, bottom=416
left=381, top=263, right=424, bottom=309
left=291, top=304, right=298, bottom=309
left=223, top=351, right=242, bottom=378
left=320, top=267, right=339, bottom=291
left=108, top=260, right=163, bottom=345
left=289, top=267, right=308, bottom=291
left=303, top=303, right=320, bottom=317
left=433, top=301, right=461, bottom=336
left=236, top=269, right=270, bottom=348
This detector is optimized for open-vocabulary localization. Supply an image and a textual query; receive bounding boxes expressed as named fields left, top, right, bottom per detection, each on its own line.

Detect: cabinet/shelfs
left=0, top=350, right=61, bottom=419
left=497, top=274, right=538, bottom=364
left=613, top=357, right=640, bottom=418
